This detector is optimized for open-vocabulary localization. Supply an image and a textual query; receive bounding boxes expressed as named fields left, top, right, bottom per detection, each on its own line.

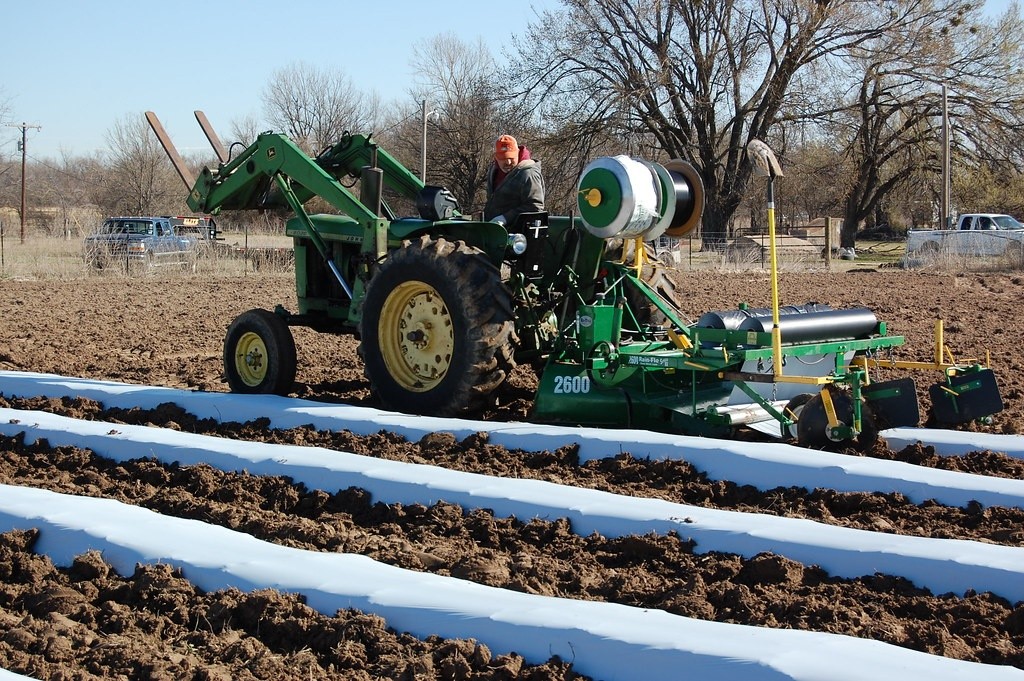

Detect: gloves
left=489, top=215, right=506, bottom=226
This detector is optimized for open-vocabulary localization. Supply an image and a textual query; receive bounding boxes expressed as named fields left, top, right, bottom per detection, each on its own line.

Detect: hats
left=495, top=135, right=519, bottom=160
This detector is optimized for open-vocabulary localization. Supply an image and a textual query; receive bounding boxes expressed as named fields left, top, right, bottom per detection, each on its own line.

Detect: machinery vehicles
left=145, top=107, right=1003, bottom=448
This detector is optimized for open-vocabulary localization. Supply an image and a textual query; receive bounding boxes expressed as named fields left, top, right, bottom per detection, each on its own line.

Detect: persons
left=479, top=135, right=546, bottom=227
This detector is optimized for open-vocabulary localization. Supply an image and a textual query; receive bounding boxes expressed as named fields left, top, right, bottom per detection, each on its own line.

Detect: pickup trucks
left=906, top=214, right=1024, bottom=269
left=84, top=215, right=197, bottom=272
